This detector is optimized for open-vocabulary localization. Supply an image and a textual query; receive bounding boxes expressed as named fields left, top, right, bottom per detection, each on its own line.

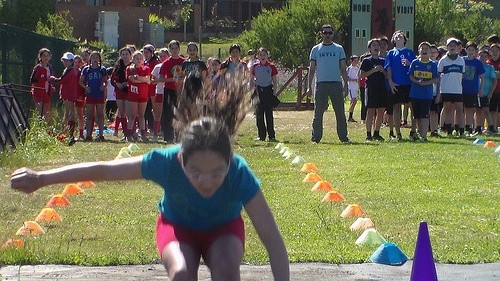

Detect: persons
left=345, top=30, right=500, bottom=143
left=30, top=48, right=55, bottom=119
left=9, top=116, right=289, bottom=281
left=49, top=39, right=260, bottom=147
left=306, top=24, right=350, bottom=145
left=250, top=46, right=281, bottom=142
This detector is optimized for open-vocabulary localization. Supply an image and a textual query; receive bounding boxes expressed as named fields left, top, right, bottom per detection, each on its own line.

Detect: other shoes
left=429, top=128, right=497, bottom=138
left=84, top=136, right=93, bottom=142
left=95, top=134, right=104, bottom=142
left=373, top=136, right=384, bottom=141
left=344, top=139, right=351, bottom=143
left=79, top=136, right=85, bottom=141
left=122, top=136, right=148, bottom=142
left=311, top=137, right=320, bottom=142
left=348, top=118, right=357, bottom=123
left=409, top=132, right=420, bottom=142
left=269, top=138, right=279, bottom=142
left=256, top=137, right=265, bottom=141
left=152, top=136, right=158, bottom=143
left=417, top=131, right=427, bottom=141
left=366, top=137, right=372, bottom=141
left=396, top=134, right=405, bottom=142
left=113, top=130, right=118, bottom=137
left=69, top=140, right=76, bottom=146
left=388, top=135, right=396, bottom=142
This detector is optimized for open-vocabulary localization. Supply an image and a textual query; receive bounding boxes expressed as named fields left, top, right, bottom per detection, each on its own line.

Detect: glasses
left=248, top=53, right=254, bottom=55
left=322, top=31, right=334, bottom=35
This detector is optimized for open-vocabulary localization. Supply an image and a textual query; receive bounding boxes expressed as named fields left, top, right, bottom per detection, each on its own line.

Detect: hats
left=61, top=52, right=75, bottom=61
